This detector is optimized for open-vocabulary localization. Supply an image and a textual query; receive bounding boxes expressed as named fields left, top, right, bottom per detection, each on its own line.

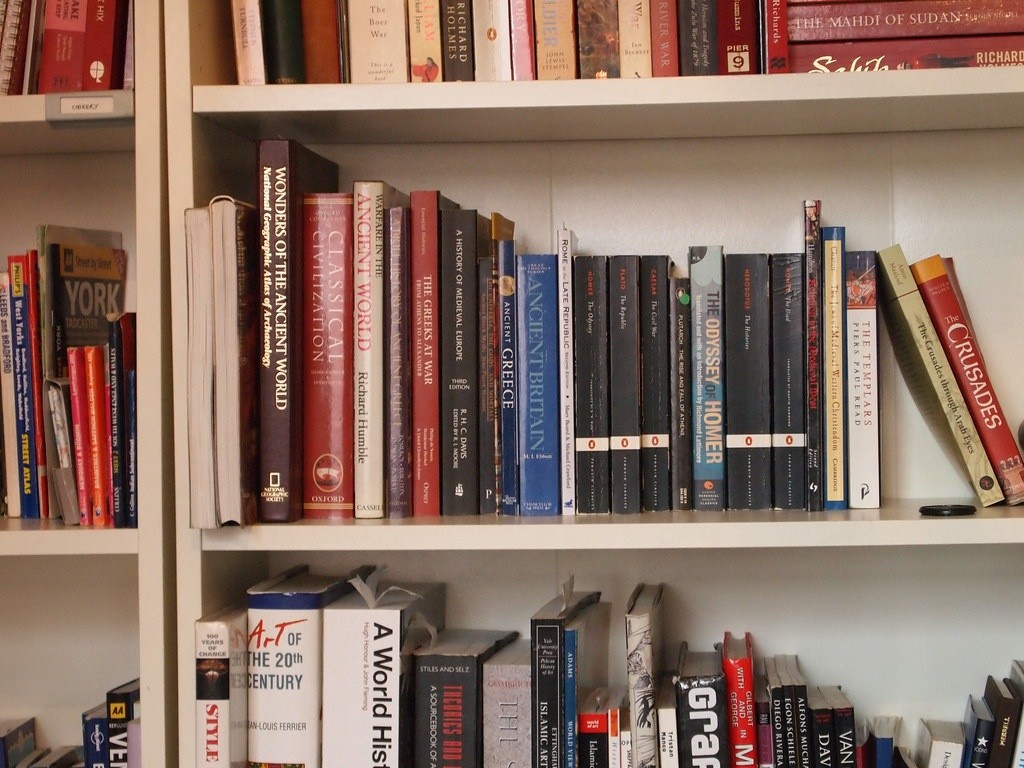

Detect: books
left=0, top=222, right=138, bottom=528
left=1, top=0, right=125, bottom=96
left=183, top=135, right=1024, bottom=528
left=229, top=0, right=1024, bottom=86
left=195, top=563, right=1024, bottom=768
left=0, top=676, right=141, bottom=768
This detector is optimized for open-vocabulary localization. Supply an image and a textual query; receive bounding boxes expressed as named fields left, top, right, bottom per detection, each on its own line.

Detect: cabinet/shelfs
left=0, top=0, right=1024, bottom=768
